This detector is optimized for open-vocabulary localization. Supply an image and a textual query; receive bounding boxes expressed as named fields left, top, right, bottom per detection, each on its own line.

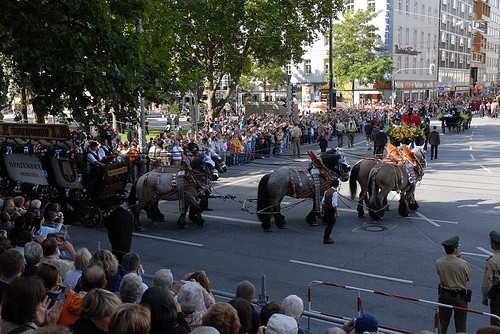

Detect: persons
left=0, top=91, right=500, bottom=212
left=320, top=179, right=340, bottom=245
left=0, top=197, right=437, bottom=334
left=476, top=231, right=500, bottom=334
left=435, top=235, right=472, bottom=334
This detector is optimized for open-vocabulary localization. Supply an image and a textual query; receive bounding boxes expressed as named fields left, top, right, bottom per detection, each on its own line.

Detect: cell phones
left=57, top=242, right=63, bottom=247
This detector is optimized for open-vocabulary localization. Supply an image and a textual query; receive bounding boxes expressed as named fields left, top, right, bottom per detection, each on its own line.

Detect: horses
left=256, top=150, right=351, bottom=231
left=349, top=137, right=429, bottom=221
left=420, top=117, right=430, bottom=139
left=464, top=107, right=472, bottom=128
left=125, top=149, right=227, bottom=230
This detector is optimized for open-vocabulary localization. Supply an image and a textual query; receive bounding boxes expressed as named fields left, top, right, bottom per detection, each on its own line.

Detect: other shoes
left=205, top=208, right=213, bottom=211
left=323, top=237, right=335, bottom=244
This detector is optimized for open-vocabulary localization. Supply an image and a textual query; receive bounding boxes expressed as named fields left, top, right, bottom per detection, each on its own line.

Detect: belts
left=440, top=286, right=463, bottom=294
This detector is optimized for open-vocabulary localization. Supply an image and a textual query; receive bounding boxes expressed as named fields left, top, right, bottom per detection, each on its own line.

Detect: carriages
left=439, top=106, right=473, bottom=135
left=0, top=122, right=428, bottom=232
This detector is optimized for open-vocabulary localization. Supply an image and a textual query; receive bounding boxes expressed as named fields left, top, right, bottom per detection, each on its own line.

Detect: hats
left=88, top=141, right=99, bottom=147
left=355, top=314, right=378, bottom=334
left=489, top=230, right=499, bottom=243
left=440, top=235, right=461, bottom=248
left=265, top=313, right=298, bottom=334
